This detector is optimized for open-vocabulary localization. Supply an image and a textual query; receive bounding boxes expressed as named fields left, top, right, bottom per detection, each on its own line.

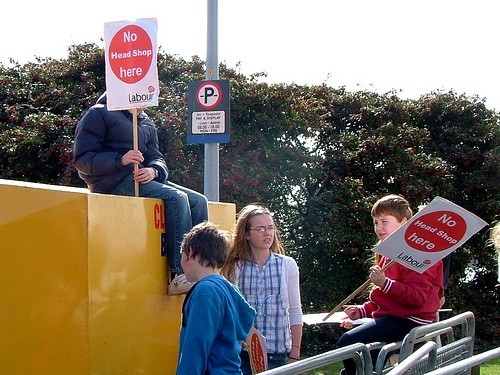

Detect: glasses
left=247, top=227, right=275, bottom=232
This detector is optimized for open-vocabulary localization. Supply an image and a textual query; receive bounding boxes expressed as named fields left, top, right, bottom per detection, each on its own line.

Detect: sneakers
left=168, top=274, right=197, bottom=296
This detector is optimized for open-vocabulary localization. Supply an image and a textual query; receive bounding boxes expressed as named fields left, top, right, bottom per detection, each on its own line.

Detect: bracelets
left=287, top=355, right=300, bottom=361
left=291, top=345, right=302, bottom=348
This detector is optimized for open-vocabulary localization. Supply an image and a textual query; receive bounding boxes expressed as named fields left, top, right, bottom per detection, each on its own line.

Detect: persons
left=338, top=193, right=444, bottom=375
left=73, top=91, right=209, bottom=295
left=219, top=205, right=304, bottom=368
left=176, top=220, right=258, bottom=375
